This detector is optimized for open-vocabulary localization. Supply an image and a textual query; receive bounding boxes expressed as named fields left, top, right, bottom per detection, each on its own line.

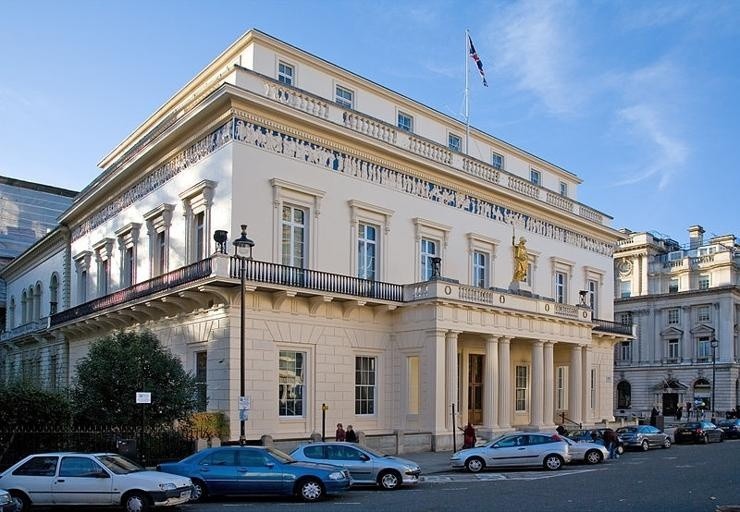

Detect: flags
left=469, top=35, right=487, bottom=87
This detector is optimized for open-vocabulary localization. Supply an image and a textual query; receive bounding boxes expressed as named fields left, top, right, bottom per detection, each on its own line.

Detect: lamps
left=48, top=300, right=58, bottom=314
left=431, top=257, right=442, bottom=277
left=212, top=229, right=229, bottom=254
left=577, top=290, right=590, bottom=309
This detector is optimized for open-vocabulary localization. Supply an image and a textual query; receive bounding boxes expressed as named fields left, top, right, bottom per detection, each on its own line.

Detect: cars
left=157, top=444, right=354, bottom=503
left=280, top=407, right=297, bottom=416
left=1, top=489, right=11, bottom=511
left=561, top=435, right=610, bottom=465
left=566, top=428, right=625, bottom=459
left=451, top=432, right=572, bottom=471
left=286, top=442, right=421, bottom=489
left=718, top=418, right=740, bottom=437
left=675, top=421, right=725, bottom=443
left=0, top=451, right=192, bottom=511
left=695, top=401, right=706, bottom=409
left=616, top=425, right=672, bottom=451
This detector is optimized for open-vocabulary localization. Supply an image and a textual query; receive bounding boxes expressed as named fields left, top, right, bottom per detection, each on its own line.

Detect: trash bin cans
left=120, top=439, right=137, bottom=464
left=651, top=416, right=664, bottom=432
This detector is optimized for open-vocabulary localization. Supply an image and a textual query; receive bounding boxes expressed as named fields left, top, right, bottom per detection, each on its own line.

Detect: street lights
left=233, top=223, right=254, bottom=445
left=709, top=334, right=720, bottom=412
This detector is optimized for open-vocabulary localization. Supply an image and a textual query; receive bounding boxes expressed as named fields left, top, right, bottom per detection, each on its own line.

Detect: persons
left=604, top=430, right=619, bottom=460
left=512, top=236, right=528, bottom=282
left=336, top=423, right=346, bottom=442
left=345, top=425, right=357, bottom=442
left=464, top=423, right=476, bottom=448
